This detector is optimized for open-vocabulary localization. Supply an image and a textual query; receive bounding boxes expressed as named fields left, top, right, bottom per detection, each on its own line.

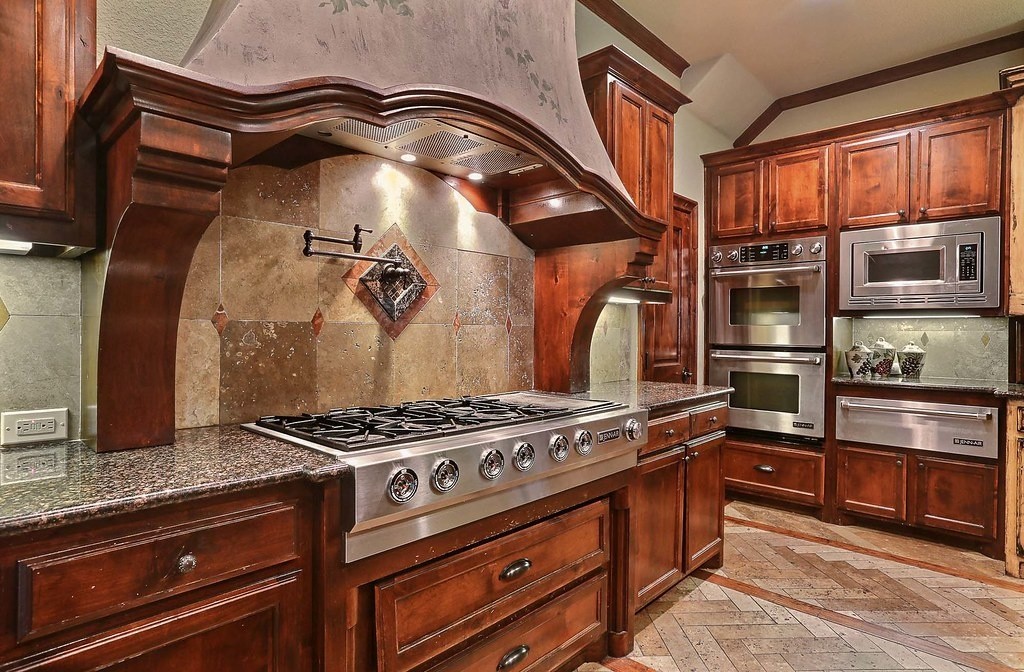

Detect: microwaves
left=840, top=217, right=1000, bottom=311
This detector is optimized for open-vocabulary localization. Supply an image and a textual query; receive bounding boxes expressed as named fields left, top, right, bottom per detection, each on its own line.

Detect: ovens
left=709, top=237, right=825, bottom=442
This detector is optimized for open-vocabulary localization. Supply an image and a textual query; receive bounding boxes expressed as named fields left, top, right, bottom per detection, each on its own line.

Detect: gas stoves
left=240, top=389, right=648, bottom=563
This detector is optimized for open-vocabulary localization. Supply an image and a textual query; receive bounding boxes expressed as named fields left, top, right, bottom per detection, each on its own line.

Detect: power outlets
left=2, top=407, right=69, bottom=446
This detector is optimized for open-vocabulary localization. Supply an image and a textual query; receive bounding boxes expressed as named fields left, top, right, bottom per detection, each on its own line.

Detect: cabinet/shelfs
left=699, top=66, right=1024, bottom=561
left=0, top=0, right=97, bottom=258
left=577, top=43, right=694, bottom=303
left=0, top=380, right=735, bottom=672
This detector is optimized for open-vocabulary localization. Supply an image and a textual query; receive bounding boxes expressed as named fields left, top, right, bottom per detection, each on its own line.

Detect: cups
left=868, top=337, right=896, bottom=377
left=845, top=340, right=873, bottom=379
left=897, top=341, right=926, bottom=378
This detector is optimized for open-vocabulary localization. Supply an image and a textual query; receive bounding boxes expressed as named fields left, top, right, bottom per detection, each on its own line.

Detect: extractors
left=298, top=117, right=547, bottom=186
